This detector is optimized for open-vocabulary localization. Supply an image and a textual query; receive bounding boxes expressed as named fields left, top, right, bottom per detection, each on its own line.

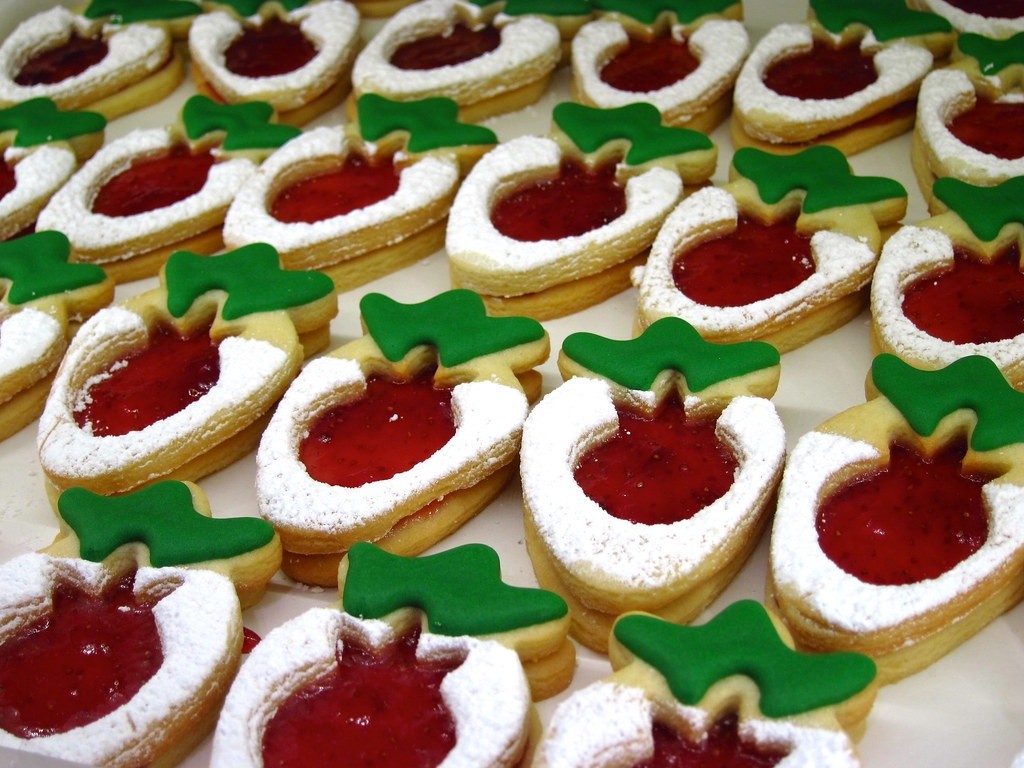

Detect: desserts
left=1, top=2, right=1024, bottom=768
